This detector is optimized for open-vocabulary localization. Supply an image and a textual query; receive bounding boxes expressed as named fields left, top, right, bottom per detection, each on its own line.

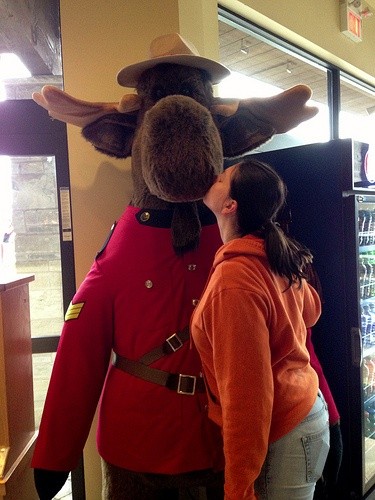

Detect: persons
left=189, top=159, right=331, bottom=500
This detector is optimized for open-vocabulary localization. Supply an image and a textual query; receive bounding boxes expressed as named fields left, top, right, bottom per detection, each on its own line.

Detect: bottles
left=358, top=250, right=375, bottom=301
left=358, top=209, right=375, bottom=246
left=360, top=304, right=375, bottom=348
left=364, top=403, right=375, bottom=440
left=362, top=354, right=375, bottom=402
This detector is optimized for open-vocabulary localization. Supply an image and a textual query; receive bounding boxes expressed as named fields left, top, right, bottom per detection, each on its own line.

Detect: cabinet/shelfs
left=1, top=268, right=44, bottom=500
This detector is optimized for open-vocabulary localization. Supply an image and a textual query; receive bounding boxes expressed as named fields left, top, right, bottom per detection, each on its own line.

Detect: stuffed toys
left=30, top=34, right=318, bottom=500
